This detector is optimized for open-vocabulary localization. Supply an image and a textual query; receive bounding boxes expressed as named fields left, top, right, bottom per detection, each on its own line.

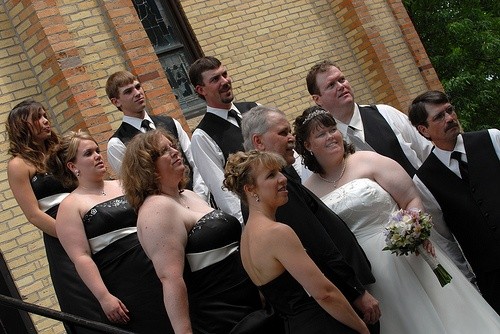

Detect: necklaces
left=316, top=159, right=346, bottom=187
left=162, top=189, right=190, bottom=209
left=78, top=183, right=106, bottom=195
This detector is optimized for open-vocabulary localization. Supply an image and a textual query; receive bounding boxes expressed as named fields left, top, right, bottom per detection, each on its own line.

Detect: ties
left=228, top=109, right=243, bottom=128
left=347, top=127, right=377, bottom=152
left=450, top=151, right=468, bottom=179
left=141, top=119, right=154, bottom=131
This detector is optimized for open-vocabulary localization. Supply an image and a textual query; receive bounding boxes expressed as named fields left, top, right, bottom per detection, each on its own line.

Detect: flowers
left=383, top=208, right=453, bottom=288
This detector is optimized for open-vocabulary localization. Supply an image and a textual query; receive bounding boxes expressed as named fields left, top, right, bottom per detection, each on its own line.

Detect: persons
left=305, top=60, right=480, bottom=296
left=292, top=104, right=445, bottom=334
left=220, top=147, right=371, bottom=333
left=409, top=90, right=499, bottom=313
left=119, top=128, right=262, bottom=334
left=188, top=57, right=267, bottom=224
left=241, top=106, right=382, bottom=334
left=45, top=131, right=174, bottom=334
left=104, top=70, right=212, bottom=206
left=4, top=98, right=112, bottom=334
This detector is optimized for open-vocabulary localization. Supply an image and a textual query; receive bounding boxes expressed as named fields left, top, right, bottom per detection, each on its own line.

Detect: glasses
left=425, top=106, right=455, bottom=122
